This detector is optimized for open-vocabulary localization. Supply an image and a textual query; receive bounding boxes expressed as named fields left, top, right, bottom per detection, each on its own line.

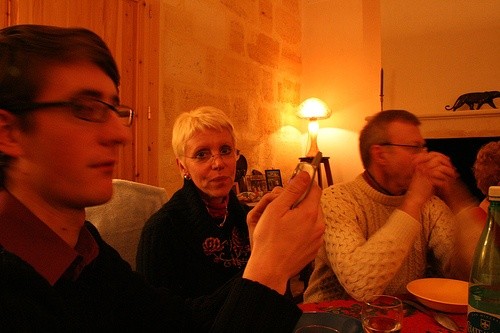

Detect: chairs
left=85, top=179, right=167, bottom=272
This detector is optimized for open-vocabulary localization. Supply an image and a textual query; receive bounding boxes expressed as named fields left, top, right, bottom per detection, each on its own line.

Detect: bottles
left=468, top=186, right=500, bottom=333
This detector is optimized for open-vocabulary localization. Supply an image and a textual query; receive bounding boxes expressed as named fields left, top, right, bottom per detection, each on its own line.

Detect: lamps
left=296, top=96, right=330, bottom=158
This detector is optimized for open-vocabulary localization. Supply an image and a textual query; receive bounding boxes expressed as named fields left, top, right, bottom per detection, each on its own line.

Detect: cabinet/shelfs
left=298, top=157, right=333, bottom=190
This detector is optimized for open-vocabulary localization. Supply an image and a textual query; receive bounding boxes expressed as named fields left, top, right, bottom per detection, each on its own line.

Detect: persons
left=0, top=23, right=327, bottom=333
left=135, top=105, right=254, bottom=285
left=470, top=140, right=500, bottom=224
left=303, top=109, right=500, bottom=304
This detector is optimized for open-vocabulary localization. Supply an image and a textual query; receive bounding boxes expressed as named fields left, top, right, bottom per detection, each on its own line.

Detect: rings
left=296, top=290, right=301, bottom=293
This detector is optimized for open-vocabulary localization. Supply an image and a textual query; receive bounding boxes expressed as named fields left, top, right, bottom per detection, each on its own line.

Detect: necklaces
left=207, top=203, right=227, bottom=227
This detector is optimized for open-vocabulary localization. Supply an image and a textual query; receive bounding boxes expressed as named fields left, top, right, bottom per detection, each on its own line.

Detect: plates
left=294, top=312, right=364, bottom=332
left=406, top=278, right=471, bottom=314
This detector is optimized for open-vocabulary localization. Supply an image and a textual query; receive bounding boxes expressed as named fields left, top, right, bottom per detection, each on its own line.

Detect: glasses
left=379, top=143, right=427, bottom=155
left=182, top=146, right=240, bottom=166
left=13, top=96, right=139, bottom=127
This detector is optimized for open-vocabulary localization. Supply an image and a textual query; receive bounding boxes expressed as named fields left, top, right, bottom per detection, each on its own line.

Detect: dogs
left=445, top=90, right=500, bottom=112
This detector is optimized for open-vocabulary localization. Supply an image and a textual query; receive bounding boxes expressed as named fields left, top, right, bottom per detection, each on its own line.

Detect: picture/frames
left=245, top=175, right=268, bottom=193
left=265, top=169, right=283, bottom=191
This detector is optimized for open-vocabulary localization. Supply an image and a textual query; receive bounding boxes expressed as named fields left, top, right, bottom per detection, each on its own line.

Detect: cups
left=360, top=295, right=403, bottom=333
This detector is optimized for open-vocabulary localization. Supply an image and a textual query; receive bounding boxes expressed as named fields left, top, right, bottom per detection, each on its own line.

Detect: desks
left=291, top=293, right=469, bottom=333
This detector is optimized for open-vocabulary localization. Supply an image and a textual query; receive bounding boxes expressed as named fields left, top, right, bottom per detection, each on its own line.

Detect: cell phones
left=292, top=152, right=323, bottom=209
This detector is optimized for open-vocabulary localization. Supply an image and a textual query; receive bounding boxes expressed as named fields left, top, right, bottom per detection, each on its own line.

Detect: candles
left=380, top=67, right=384, bottom=96
